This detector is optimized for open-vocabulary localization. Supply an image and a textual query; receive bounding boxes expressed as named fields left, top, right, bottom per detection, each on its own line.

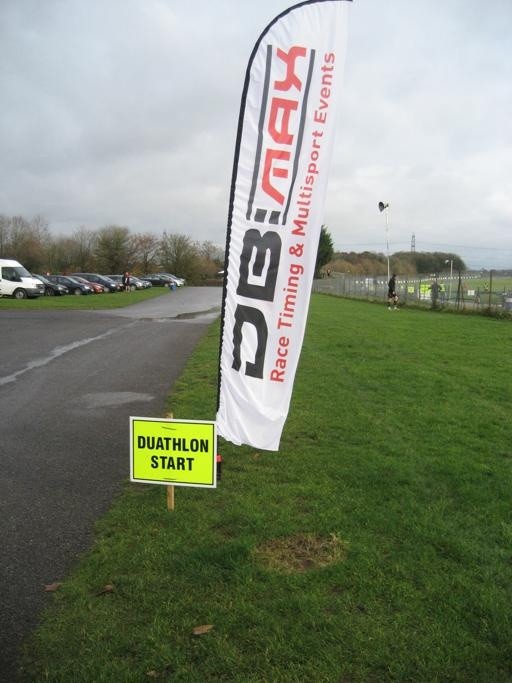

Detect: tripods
left=455, top=271, right=466, bottom=311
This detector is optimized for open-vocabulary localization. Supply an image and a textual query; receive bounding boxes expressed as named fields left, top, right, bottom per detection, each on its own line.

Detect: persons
left=386, top=272, right=401, bottom=312
left=427, top=278, right=443, bottom=307
left=439, top=280, right=447, bottom=304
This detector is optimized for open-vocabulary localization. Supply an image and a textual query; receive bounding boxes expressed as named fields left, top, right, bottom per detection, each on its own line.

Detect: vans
left=0, top=255, right=47, bottom=302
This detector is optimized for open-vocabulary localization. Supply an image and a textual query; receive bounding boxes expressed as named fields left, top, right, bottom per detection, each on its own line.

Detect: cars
left=32, top=272, right=186, bottom=296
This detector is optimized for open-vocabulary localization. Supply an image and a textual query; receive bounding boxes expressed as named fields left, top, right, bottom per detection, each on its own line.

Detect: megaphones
left=379, top=202, right=388, bottom=211
